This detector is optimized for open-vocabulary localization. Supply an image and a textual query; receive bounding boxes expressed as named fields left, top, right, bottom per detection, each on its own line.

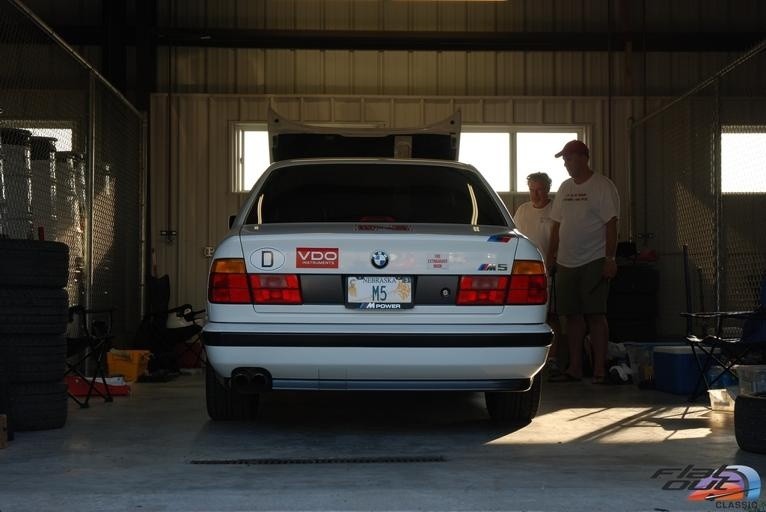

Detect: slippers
left=547, top=373, right=584, bottom=383
left=592, top=376, right=604, bottom=384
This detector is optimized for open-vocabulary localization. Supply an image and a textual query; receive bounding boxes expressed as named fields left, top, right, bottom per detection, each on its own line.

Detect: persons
left=546, top=140, right=621, bottom=385
left=512, top=172, right=554, bottom=318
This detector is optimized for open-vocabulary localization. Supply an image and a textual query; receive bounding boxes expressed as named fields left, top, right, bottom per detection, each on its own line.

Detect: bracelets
left=606, top=255, right=616, bottom=261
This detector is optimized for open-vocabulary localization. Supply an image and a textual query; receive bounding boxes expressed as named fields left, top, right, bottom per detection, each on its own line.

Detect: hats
left=555, top=140, right=589, bottom=159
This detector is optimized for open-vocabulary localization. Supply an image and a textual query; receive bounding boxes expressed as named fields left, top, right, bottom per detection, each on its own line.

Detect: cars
left=192, top=156, right=558, bottom=425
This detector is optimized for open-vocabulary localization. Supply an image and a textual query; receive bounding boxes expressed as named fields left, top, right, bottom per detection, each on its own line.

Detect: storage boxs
left=653, top=347, right=724, bottom=394
left=108, top=348, right=151, bottom=382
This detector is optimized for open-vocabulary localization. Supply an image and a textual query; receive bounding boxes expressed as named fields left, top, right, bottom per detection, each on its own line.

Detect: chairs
left=687, top=306, right=766, bottom=406
left=63, top=288, right=120, bottom=408
